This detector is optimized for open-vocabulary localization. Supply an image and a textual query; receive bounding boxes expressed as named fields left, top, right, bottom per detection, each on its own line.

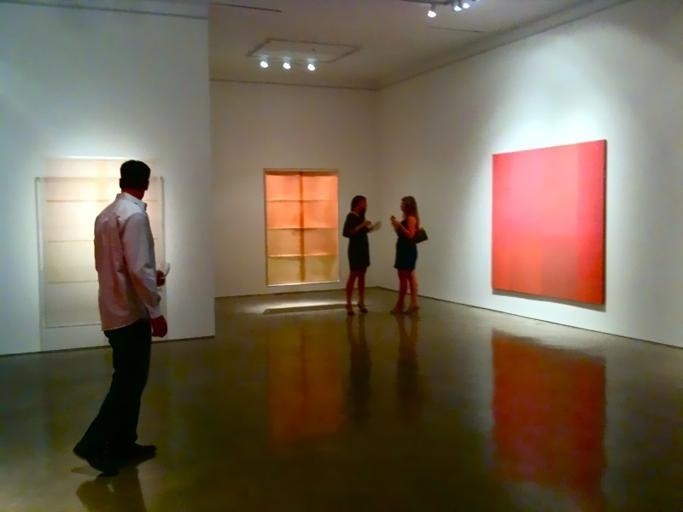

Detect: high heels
left=345, top=302, right=368, bottom=316
left=389, top=305, right=419, bottom=315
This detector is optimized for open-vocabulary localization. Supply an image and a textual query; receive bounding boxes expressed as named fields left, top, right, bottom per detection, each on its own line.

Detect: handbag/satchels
left=414, top=229, right=427, bottom=243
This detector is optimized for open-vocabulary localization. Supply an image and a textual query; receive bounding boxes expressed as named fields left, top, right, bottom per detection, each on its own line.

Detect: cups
left=160, top=262, right=170, bottom=276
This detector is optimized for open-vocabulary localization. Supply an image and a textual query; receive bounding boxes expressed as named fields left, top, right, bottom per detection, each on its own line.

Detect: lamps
left=427, top=0, right=471, bottom=18
left=259, top=54, right=318, bottom=71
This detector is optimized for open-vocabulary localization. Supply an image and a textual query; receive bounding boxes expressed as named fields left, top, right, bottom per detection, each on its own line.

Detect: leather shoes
left=73, top=442, right=156, bottom=474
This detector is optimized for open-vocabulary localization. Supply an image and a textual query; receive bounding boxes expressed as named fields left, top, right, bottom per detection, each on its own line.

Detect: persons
left=390, top=196, right=420, bottom=315
left=342, top=195, right=375, bottom=314
left=72, top=159, right=166, bottom=476
